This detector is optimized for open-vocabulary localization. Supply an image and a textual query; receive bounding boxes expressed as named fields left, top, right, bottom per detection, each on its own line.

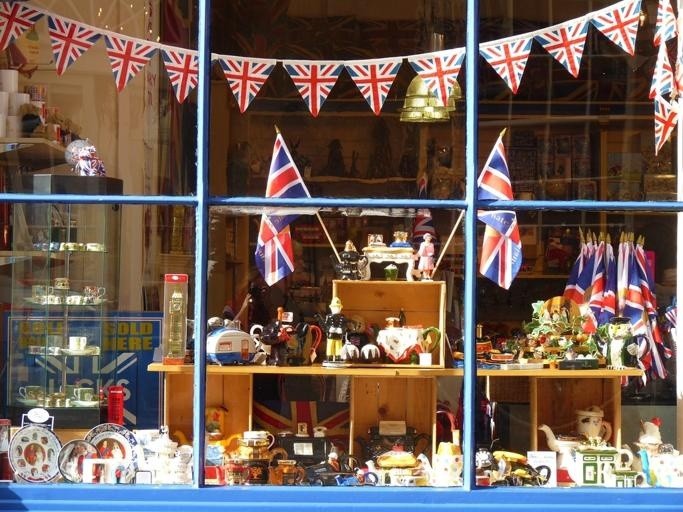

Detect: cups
left=244, top=430, right=275, bottom=449
left=624, top=335, right=650, bottom=367
left=0, top=69, right=105, bottom=406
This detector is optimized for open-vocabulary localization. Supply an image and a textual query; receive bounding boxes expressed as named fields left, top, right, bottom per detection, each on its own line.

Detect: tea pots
left=366, top=424, right=683, bottom=488
left=372, top=324, right=441, bottom=364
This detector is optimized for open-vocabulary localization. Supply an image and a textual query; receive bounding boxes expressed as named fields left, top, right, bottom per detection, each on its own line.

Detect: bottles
left=608, top=317, right=635, bottom=366
left=0, top=419, right=11, bottom=451
left=575, top=410, right=603, bottom=438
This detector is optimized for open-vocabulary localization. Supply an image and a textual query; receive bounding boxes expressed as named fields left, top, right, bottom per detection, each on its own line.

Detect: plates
left=8, top=424, right=138, bottom=484
left=87, top=299, right=108, bottom=306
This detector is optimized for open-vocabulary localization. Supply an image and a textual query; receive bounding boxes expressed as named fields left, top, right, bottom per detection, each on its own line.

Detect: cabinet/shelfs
left=146, top=78, right=677, bottom=464
left=0, top=136, right=123, bottom=428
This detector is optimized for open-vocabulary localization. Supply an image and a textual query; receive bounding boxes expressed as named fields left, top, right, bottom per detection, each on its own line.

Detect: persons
left=259, top=295, right=369, bottom=370
left=414, top=232, right=435, bottom=282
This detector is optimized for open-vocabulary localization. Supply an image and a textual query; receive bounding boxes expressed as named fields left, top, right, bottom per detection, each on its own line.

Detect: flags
left=254, top=134, right=309, bottom=288
left=413, top=174, right=440, bottom=269
left=476, top=136, right=523, bottom=290
left=563, top=224, right=673, bottom=391
